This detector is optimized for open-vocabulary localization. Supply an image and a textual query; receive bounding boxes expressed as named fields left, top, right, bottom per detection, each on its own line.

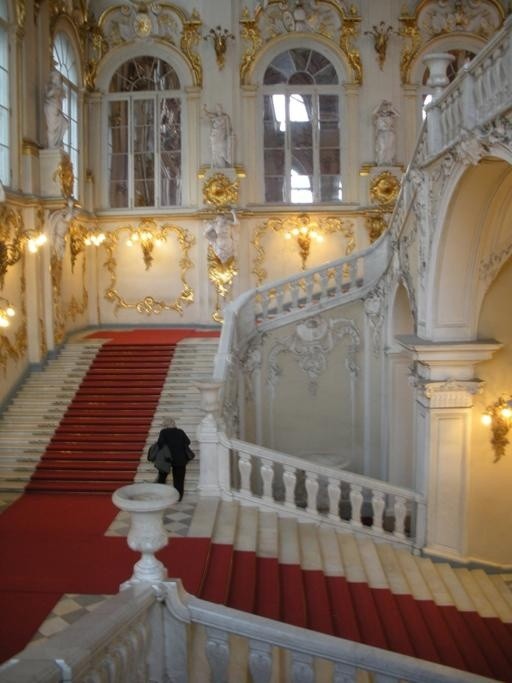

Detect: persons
left=202, top=102, right=232, bottom=168
left=148, top=417, right=195, bottom=502
left=373, top=100, right=399, bottom=167
left=43, top=71, right=69, bottom=150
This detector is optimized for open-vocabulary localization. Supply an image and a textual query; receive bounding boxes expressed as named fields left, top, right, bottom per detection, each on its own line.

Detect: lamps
left=202, top=25, right=237, bottom=71
left=479, top=391, right=512, bottom=464
left=0, top=297, right=16, bottom=329
left=20, top=228, right=48, bottom=253
left=284, top=225, right=324, bottom=270
left=364, top=20, right=401, bottom=71
left=81, top=221, right=106, bottom=247
left=124, top=229, right=163, bottom=271
